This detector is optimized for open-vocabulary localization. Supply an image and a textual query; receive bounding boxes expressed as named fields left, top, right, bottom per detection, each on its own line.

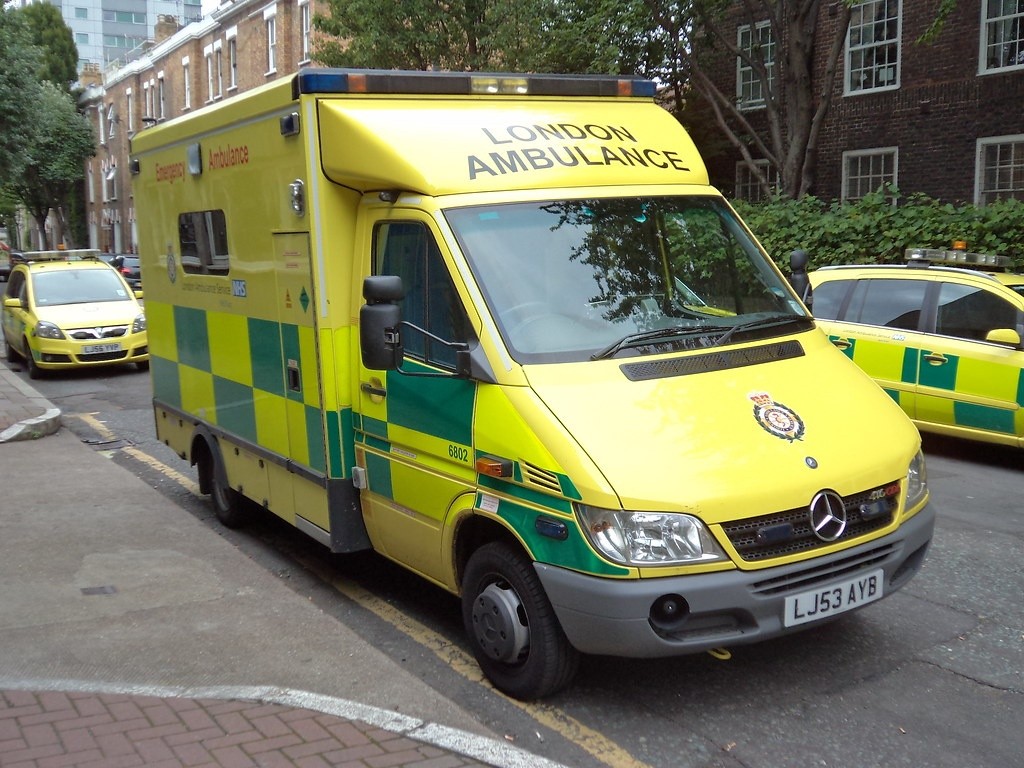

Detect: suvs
left=98, top=252, right=142, bottom=292
left=806, top=241, right=1024, bottom=449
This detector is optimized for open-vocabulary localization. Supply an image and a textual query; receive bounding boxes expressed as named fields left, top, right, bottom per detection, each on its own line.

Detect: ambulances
left=127, top=65, right=937, bottom=703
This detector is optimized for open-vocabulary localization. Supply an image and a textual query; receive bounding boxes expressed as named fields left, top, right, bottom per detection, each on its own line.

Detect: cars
left=0, top=249, right=148, bottom=379
left=0, top=249, right=12, bottom=282
